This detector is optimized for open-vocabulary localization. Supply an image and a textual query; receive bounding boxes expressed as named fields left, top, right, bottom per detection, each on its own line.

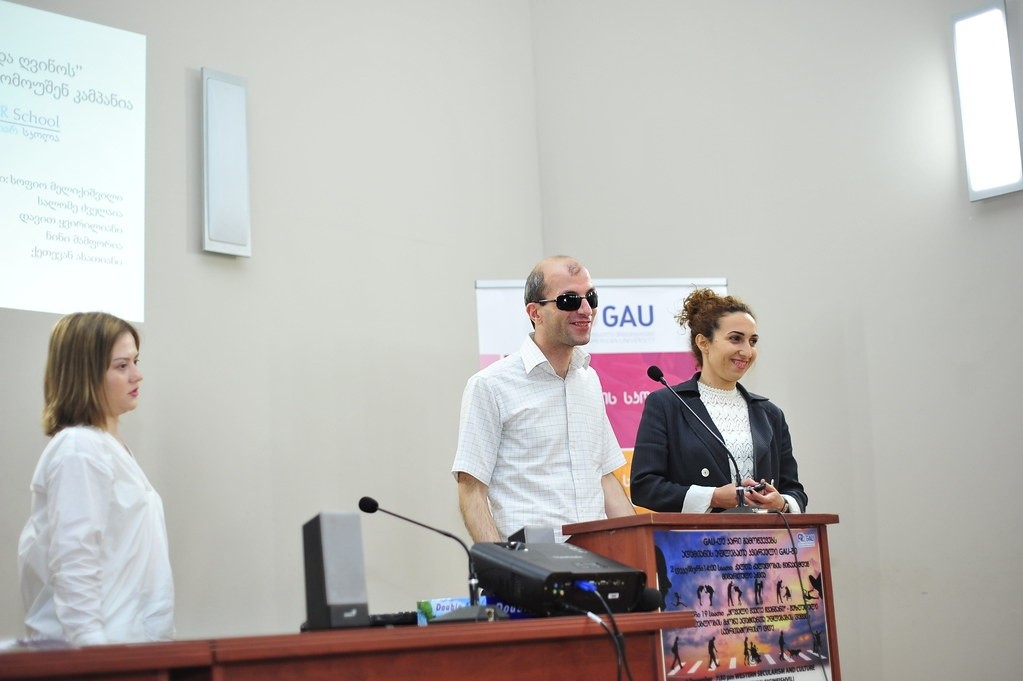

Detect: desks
left=0, top=611, right=696, bottom=681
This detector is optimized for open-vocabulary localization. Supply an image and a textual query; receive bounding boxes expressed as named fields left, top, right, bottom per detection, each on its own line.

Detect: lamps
left=950, top=1, right=1023, bottom=203
left=200, top=66, right=252, bottom=257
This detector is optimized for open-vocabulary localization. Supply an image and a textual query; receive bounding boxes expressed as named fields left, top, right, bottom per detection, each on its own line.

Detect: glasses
left=532, top=288, right=598, bottom=312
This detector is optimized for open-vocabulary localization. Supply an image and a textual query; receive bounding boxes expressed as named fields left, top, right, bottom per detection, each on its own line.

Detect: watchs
left=781, top=500, right=789, bottom=513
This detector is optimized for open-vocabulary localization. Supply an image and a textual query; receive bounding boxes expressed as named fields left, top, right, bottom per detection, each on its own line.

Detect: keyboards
left=369, top=612, right=418, bottom=627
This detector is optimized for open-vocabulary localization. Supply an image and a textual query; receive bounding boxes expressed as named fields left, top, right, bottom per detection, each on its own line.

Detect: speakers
left=508, top=524, right=556, bottom=545
left=299, top=512, right=371, bottom=628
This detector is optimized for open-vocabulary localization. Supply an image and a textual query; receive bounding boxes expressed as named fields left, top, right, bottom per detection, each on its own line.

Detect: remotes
left=753, top=484, right=766, bottom=492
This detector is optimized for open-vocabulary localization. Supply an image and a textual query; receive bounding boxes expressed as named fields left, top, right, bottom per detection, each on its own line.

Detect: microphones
left=359, top=497, right=510, bottom=622
left=647, top=365, right=763, bottom=514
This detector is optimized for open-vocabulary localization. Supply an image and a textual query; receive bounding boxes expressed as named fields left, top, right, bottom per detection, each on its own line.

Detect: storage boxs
left=418, top=595, right=534, bottom=627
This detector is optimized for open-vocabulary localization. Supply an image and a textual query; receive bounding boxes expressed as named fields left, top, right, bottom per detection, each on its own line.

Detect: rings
left=744, top=503, right=749, bottom=507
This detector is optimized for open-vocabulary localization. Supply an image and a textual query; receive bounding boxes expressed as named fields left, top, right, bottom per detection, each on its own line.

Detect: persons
left=630, top=288, right=808, bottom=514
left=452, top=256, right=635, bottom=545
left=17, top=312, right=176, bottom=648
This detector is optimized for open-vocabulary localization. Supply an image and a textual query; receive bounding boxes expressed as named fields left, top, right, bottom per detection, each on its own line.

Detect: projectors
left=470, top=541, right=647, bottom=618
